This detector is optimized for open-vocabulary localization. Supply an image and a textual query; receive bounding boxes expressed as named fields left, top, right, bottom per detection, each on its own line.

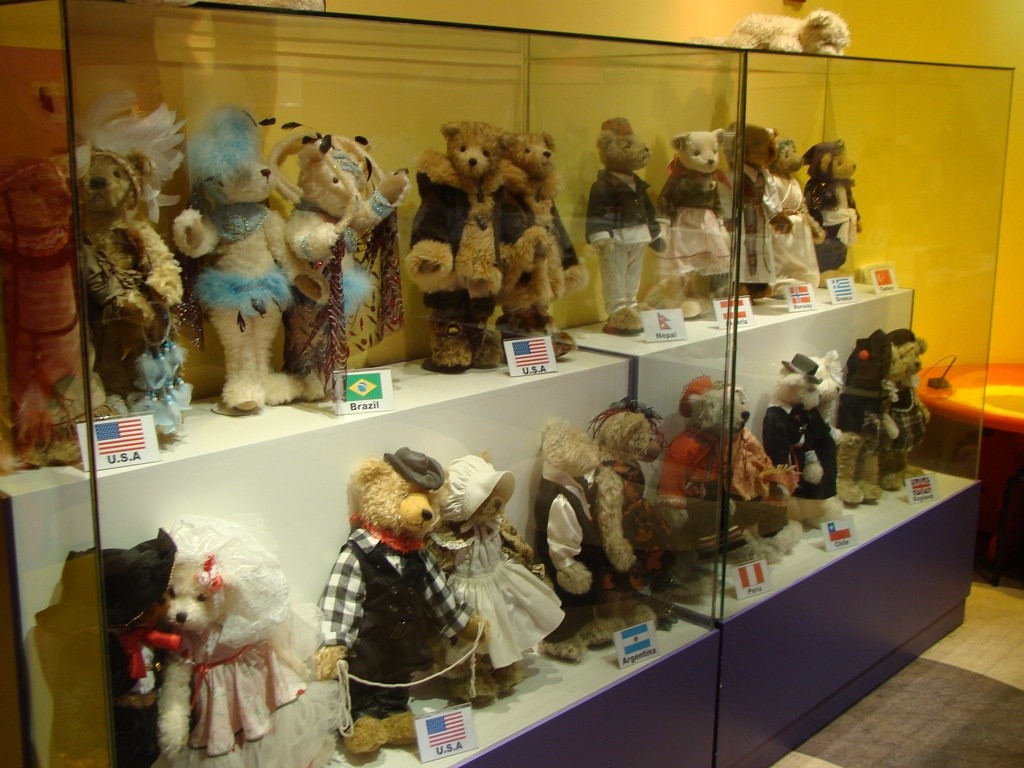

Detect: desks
left=913, top=361, right=1024, bottom=588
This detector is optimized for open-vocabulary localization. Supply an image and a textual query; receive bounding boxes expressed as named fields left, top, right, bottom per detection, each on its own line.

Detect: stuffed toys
left=761, top=350, right=844, bottom=530
left=313, top=447, right=486, bottom=754
left=802, top=139, right=864, bottom=288
left=533, top=396, right=702, bottom=660
left=424, top=455, right=566, bottom=708
left=723, top=10, right=851, bottom=56
left=404, top=122, right=589, bottom=372
left=35, top=515, right=346, bottom=768
left=654, top=375, right=803, bottom=576
left=769, top=138, right=826, bottom=300
left=0, top=102, right=184, bottom=468
left=172, top=107, right=411, bottom=409
left=652, top=127, right=731, bottom=318
left=585, top=117, right=667, bottom=336
left=724, top=121, right=793, bottom=304
left=836, top=328, right=931, bottom=504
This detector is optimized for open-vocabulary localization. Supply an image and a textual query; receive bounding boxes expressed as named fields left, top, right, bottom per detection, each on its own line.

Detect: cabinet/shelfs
left=0, top=282, right=980, bottom=767
